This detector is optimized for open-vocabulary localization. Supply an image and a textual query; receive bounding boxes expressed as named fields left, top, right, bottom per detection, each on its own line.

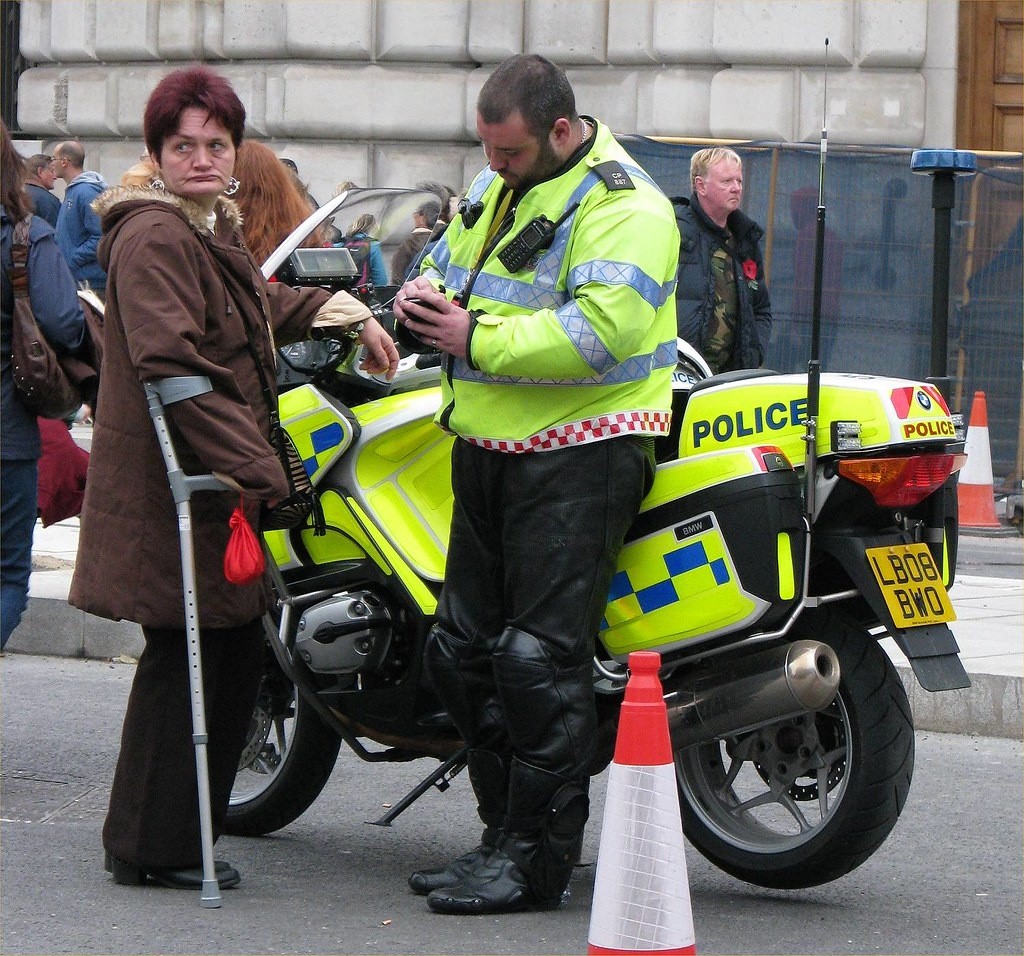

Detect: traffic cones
left=953, top=390, right=1003, bottom=528
left=584, top=646, right=700, bottom=956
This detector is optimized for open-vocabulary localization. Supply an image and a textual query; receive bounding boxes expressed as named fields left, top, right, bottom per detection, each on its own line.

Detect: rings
left=432, top=338, right=437, bottom=348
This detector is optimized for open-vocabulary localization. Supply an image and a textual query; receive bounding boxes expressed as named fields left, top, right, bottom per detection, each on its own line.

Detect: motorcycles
left=213, top=183, right=976, bottom=892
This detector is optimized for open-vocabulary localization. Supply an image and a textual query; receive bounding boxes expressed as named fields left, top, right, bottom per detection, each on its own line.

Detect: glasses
left=51, top=157, right=70, bottom=161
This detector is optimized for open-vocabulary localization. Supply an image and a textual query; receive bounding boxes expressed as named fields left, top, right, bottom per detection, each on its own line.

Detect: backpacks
left=340, top=234, right=378, bottom=286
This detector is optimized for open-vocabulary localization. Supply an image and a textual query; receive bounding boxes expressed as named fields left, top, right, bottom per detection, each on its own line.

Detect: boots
left=427, top=752, right=589, bottom=914
left=406, top=747, right=509, bottom=893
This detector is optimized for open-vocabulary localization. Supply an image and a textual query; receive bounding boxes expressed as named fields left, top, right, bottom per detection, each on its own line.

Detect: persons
left=66, top=71, right=402, bottom=891
left=0, top=116, right=88, bottom=658
left=396, top=55, right=683, bottom=917
left=668, top=147, right=774, bottom=378
left=224, top=141, right=457, bottom=291
left=50, top=139, right=110, bottom=306
left=21, top=151, right=61, bottom=228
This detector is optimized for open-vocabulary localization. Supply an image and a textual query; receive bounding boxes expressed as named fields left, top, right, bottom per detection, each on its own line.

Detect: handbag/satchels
left=11, top=295, right=94, bottom=419
left=263, top=428, right=326, bottom=537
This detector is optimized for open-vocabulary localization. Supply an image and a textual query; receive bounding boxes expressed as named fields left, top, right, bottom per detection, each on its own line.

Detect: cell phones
left=402, top=297, right=442, bottom=339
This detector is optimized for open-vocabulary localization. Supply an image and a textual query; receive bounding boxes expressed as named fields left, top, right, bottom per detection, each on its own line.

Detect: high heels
left=102, top=849, right=229, bottom=876
left=112, top=852, right=238, bottom=889
